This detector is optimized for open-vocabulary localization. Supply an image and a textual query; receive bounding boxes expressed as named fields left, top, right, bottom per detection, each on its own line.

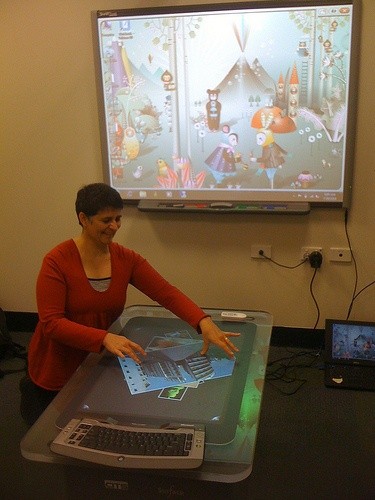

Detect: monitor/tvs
left=55, top=316, right=257, bottom=445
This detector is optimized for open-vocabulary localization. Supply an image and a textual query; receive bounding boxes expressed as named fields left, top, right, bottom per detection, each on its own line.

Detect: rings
left=224, top=338, right=228, bottom=340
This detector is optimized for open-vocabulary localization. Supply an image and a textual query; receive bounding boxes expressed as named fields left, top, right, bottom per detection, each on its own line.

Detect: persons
left=19, top=182, right=240, bottom=427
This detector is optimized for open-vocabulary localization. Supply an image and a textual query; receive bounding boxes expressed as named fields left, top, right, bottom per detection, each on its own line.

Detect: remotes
left=221, top=312, right=247, bottom=321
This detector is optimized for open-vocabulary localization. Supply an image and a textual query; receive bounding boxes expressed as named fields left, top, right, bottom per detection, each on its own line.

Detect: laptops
left=324, top=319, right=375, bottom=391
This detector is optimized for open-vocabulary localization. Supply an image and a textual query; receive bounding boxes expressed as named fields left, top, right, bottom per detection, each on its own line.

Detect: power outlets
left=300, top=247, right=323, bottom=260
left=250, top=245, right=271, bottom=258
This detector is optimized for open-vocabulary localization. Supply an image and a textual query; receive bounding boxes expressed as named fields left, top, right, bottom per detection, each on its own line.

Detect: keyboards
left=49, top=417, right=206, bottom=467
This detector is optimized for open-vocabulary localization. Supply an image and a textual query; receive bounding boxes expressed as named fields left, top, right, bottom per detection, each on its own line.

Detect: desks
left=20, top=304, right=274, bottom=499
